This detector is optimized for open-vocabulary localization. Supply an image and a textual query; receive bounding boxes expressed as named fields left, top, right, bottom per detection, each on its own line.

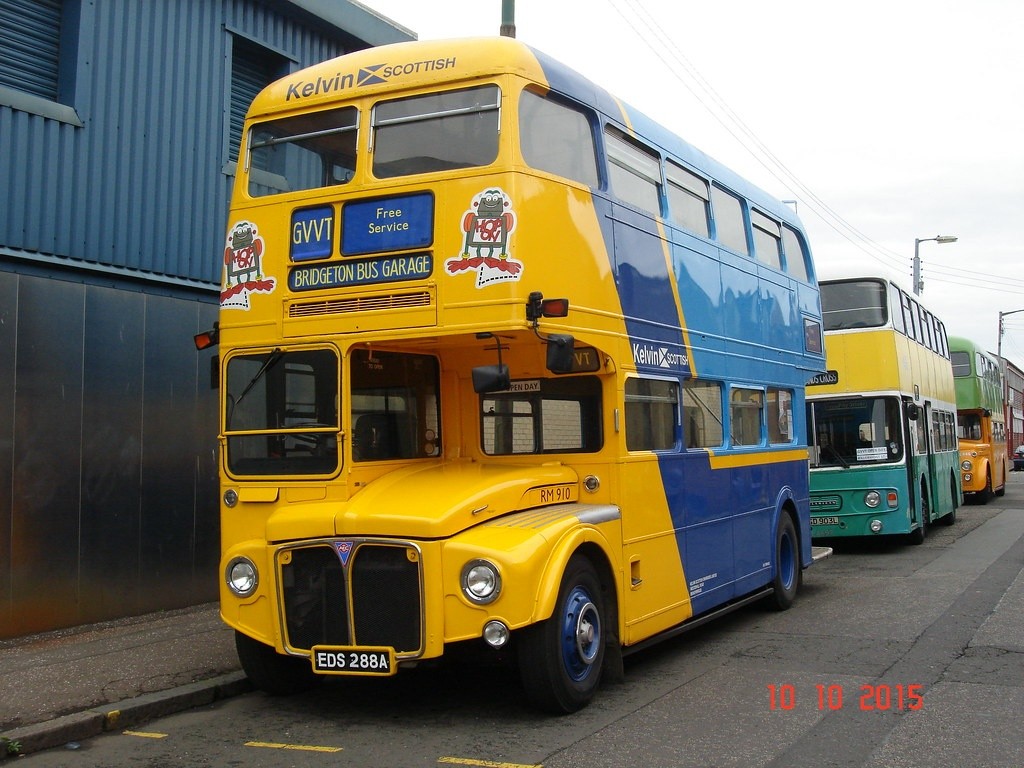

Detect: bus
left=805, top=262, right=963, bottom=550
left=193, top=33, right=834, bottom=718
left=950, top=333, right=1011, bottom=503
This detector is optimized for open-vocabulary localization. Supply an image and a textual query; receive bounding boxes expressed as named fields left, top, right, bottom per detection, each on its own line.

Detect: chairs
left=356, top=409, right=418, bottom=463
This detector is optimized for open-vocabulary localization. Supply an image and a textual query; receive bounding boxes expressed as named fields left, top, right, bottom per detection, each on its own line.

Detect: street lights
left=912, top=235, right=958, bottom=295
left=996, top=306, right=1021, bottom=359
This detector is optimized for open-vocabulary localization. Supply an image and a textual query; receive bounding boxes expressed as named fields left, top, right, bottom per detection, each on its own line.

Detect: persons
left=859, top=430, right=867, bottom=441
